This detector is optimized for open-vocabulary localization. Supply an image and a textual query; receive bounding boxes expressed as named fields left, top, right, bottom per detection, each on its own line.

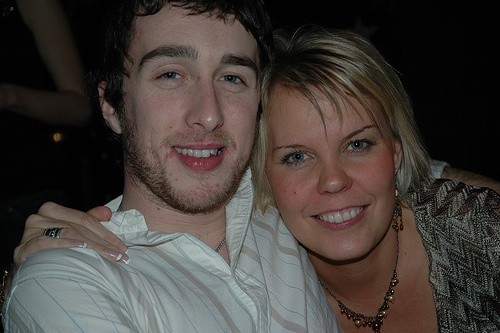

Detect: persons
left=0, top=0, right=500, bottom=333
left=13, top=24, right=500, bottom=333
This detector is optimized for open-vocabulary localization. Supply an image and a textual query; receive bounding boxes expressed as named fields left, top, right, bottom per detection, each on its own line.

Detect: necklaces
left=215, top=237, right=226, bottom=252
left=318, top=232, right=399, bottom=333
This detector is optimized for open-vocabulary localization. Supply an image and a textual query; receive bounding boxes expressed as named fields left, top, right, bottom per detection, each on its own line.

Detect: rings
left=43, top=227, right=63, bottom=239
